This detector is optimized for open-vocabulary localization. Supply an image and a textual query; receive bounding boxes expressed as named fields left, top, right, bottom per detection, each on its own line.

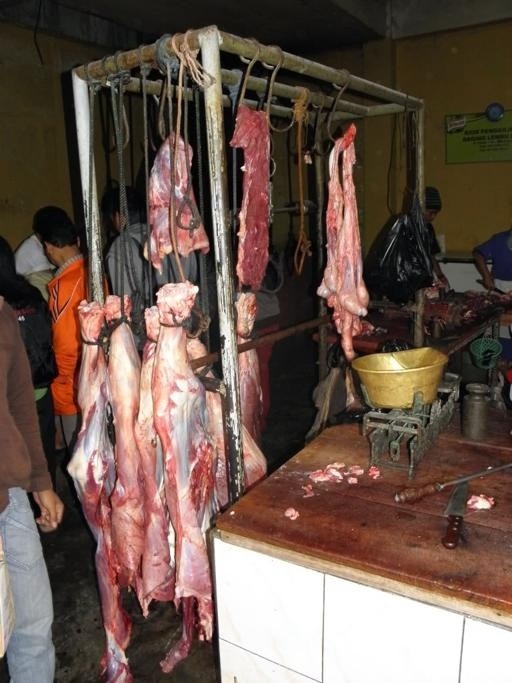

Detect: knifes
left=442, top=475, right=469, bottom=548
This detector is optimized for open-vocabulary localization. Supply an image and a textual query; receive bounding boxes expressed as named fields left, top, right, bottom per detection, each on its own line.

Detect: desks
left=313, top=299, right=507, bottom=387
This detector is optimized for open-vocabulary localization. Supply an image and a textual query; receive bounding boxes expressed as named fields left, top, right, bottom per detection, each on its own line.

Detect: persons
left=200, top=247, right=282, bottom=443
left=2, top=298, right=64, bottom=679
left=32, top=207, right=89, bottom=447
left=14, top=235, right=57, bottom=275
left=361, top=182, right=452, bottom=308
left=471, top=223, right=512, bottom=362
left=99, top=180, right=205, bottom=364
left=2, top=236, right=59, bottom=518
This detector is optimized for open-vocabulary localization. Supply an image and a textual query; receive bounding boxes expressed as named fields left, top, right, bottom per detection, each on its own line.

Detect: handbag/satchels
left=0, top=537, right=15, bottom=657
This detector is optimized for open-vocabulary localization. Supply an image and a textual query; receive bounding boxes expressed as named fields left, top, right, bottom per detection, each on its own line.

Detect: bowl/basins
left=352, top=346, right=450, bottom=409
